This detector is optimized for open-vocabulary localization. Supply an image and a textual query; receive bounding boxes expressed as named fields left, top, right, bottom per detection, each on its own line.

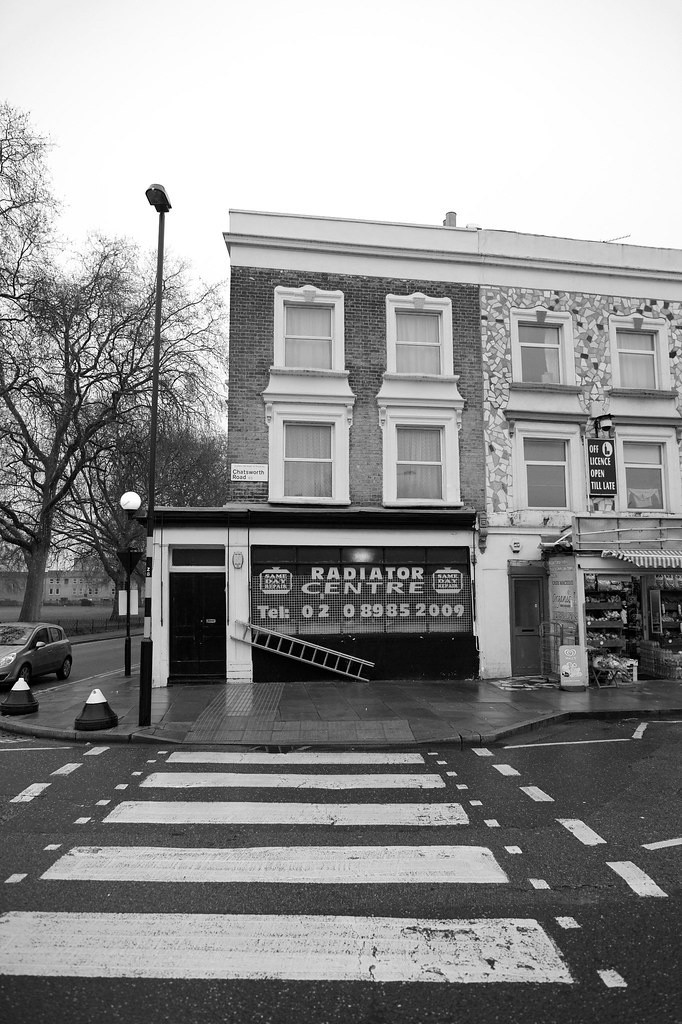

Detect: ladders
left=231, top=619, right=375, bottom=682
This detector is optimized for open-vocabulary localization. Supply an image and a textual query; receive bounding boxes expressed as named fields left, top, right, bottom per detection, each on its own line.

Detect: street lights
left=139, top=182, right=173, bottom=726
left=115, top=547, right=144, bottom=677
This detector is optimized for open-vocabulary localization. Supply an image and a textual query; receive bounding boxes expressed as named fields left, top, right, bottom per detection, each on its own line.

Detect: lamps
left=232, top=551, right=245, bottom=570
left=120, top=492, right=148, bottom=529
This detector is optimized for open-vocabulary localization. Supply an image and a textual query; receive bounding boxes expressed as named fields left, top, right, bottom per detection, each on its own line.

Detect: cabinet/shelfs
left=585, top=601, right=627, bottom=650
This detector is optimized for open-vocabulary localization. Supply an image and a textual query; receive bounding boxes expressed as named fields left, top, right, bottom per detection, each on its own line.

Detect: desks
left=590, top=668, right=624, bottom=689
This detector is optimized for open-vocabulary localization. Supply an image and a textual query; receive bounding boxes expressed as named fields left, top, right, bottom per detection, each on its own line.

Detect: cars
left=0, top=622, right=72, bottom=689
